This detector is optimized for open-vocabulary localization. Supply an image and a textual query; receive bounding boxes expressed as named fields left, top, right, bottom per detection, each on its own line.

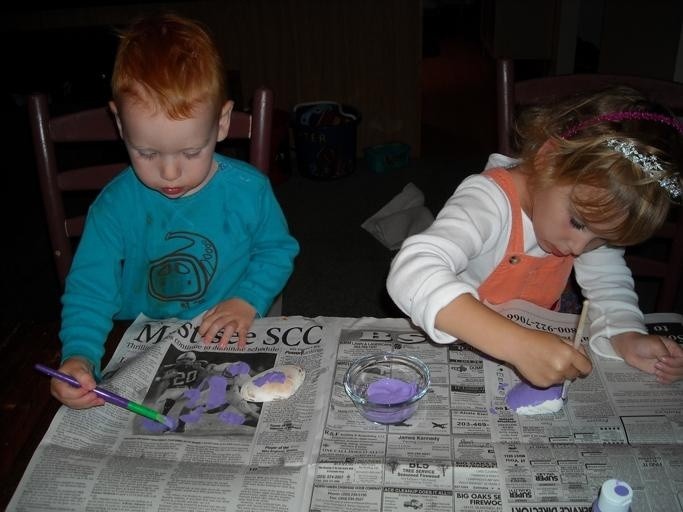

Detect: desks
left=0, top=318, right=683, bottom=512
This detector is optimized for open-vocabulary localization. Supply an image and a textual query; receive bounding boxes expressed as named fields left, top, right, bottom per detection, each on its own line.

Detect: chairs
left=495, top=59, right=683, bottom=312
left=31, top=89, right=272, bottom=289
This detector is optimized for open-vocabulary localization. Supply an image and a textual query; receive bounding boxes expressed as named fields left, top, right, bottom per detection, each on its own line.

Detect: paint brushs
left=560, top=300, right=590, bottom=401
left=35, top=364, right=176, bottom=429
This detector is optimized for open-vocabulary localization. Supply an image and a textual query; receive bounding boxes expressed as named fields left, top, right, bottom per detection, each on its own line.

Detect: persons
left=50, top=17, right=299, bottom=410
left=386, top=88, right=682, bottom=388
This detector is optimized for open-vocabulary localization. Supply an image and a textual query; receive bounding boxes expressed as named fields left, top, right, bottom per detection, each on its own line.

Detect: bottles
left=587, top=479, right=633, bottom=512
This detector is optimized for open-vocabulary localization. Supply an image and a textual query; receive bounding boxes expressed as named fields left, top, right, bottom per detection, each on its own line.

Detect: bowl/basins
left=342, top=351, right=431, bottom=424
